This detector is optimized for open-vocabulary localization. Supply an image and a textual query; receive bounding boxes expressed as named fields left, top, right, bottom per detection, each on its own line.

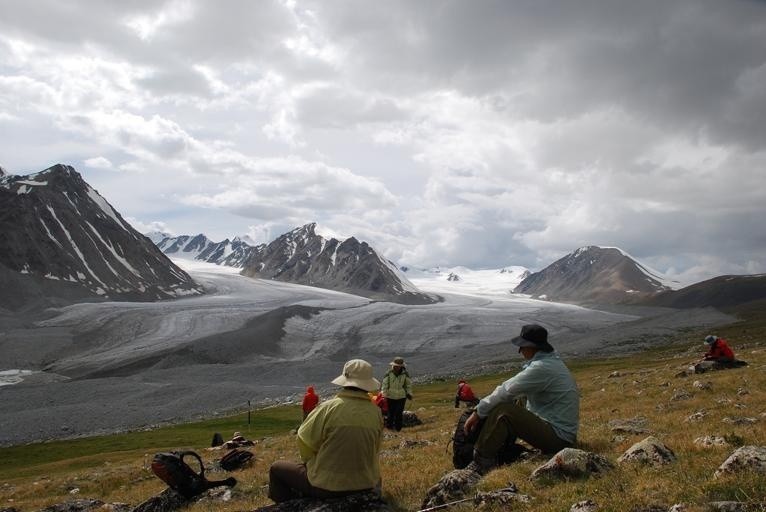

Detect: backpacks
left=220, top=449, right=253, bottom=471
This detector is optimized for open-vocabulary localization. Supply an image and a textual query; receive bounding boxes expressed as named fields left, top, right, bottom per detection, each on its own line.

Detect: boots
left=465, top=449, right=490, bottom=476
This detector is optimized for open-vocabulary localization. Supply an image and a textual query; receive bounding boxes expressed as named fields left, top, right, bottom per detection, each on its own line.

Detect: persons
left=453, top=379, right=474, bottom=408
left=701, top=334, right=737, bottom=369
left=268, top=359, right=384, bottom=505
left=462, top=324, right=582, bottom=476
left=301, top=386, right=318, bottom=421
left=381, top=356, right=412, bottom=431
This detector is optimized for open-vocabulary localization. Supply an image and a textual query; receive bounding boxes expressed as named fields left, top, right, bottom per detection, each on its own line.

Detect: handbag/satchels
left=151, top=450, right=209, bottom=500
left=453, top=410, right=480, bottom=470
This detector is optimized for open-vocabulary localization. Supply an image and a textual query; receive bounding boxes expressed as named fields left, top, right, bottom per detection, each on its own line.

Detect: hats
left=703, top=334, right=718, bottom=346
left=511, top=324, right=554, bottom=352
left=232, top=432, right=243, bottom=441
left=389, top=357, right=407, bottom=367
left=331, top=359, right=381, bottom=392
